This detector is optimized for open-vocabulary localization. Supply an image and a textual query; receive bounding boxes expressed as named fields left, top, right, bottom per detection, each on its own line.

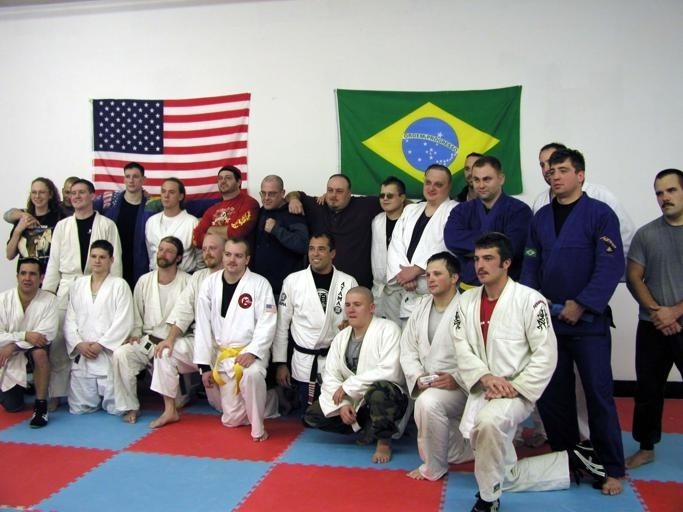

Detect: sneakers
left=572, top=440, right=608, bottom=490
left=471, top=491, right=500, bottom=512
left=514, top=433, right=546, bottom=449
left=30, top=399, right=49, bottom=429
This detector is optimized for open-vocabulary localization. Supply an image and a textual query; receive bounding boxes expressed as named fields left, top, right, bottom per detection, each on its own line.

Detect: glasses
left=259, top=191, right=281, bottom=197
left=378, top=192, right=397, bottom=199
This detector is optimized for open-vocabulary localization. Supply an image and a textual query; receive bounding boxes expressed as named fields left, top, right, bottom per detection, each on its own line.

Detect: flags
left=335, top=84, right=524, bottom=200
left=89, top=92, right=252, bottom=202
